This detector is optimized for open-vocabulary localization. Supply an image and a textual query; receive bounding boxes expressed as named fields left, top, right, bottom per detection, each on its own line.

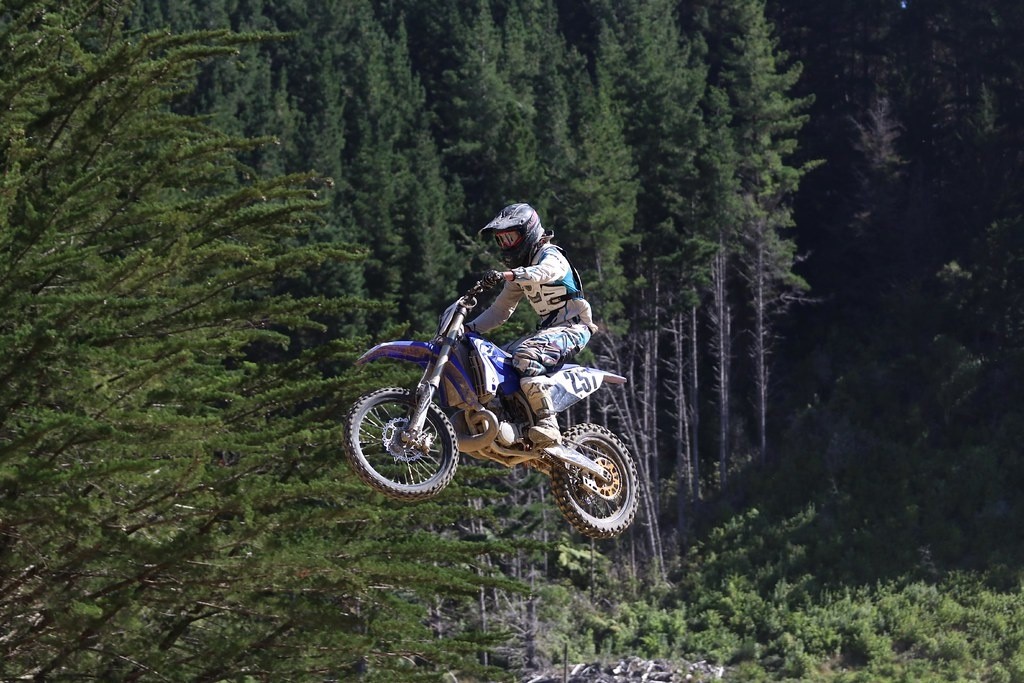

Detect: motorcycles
left=341, top=273, right=641, bottom=540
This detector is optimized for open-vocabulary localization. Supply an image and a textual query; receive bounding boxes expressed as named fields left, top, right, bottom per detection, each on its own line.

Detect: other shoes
left=528, top=408, right=562, bottom=446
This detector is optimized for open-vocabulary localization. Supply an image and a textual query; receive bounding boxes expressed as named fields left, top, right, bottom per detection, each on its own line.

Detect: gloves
left=476, top=269, right=505, bottom=296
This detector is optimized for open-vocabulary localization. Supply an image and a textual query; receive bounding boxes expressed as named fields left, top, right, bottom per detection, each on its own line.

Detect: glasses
left=491, top=229, right=521, bottom=251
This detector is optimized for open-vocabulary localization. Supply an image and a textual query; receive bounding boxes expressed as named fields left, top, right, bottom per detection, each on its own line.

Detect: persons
left=433, top=203, right=597, bottom=446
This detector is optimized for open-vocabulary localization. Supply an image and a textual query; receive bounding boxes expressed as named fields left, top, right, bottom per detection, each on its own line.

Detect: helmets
left=479, top=203, right=547, bottom=269
